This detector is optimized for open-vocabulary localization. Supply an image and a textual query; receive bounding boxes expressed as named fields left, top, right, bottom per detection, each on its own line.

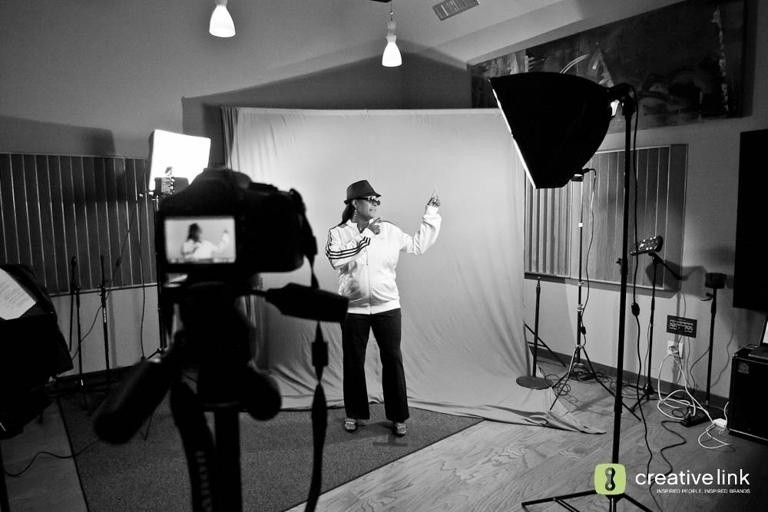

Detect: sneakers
left=343, top=417, right=357, bottom=431
left=393, top=422, right=406, bottom=436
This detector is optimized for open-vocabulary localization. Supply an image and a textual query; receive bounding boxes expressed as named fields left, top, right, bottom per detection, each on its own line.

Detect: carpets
left=50, top=364, right=485, bottom=512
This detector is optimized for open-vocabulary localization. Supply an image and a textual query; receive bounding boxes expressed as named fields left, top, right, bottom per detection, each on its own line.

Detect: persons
left=327, top=179, right=442, bottom=439
left=181, top=221, right=229, bottom=264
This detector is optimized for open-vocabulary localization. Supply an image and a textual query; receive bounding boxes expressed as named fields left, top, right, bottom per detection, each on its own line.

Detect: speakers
left=726, top=343, right=768, bottom=446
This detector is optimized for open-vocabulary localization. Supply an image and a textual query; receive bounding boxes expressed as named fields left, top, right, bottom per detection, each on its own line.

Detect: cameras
left=154, top=165, right=307, bottom=277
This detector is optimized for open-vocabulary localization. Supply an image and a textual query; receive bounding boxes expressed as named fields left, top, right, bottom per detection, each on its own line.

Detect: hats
left=343, top=179, right=381, bottom=204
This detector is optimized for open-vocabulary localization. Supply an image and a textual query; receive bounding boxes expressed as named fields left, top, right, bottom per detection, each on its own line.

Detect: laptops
left=748, top=316, right=768, bottom=360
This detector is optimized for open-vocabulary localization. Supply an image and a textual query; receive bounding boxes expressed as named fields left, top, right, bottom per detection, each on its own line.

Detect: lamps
left=142, top=128, right=213, bottom=440
left=208, top=0, right=236, bottom=38
left=381, top=0, right=403, bottom=68
left=487, top=70, right=656, bottom=512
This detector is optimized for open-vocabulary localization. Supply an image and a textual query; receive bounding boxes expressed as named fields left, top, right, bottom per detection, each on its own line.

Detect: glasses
left=358, top=197, right=379, bottom=205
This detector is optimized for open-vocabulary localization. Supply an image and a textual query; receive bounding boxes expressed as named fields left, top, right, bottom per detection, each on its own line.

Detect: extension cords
left=679, top=411, right=713, bottom=427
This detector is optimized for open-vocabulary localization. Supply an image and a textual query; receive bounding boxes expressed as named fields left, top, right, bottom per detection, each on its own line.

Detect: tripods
left=521, top=112, right=653, bottom=512
left=547, top=181, right=642, bottom=423
left=625, top=253, right=681, bottom=412
left=36, top=255, right=119, bottom=425
